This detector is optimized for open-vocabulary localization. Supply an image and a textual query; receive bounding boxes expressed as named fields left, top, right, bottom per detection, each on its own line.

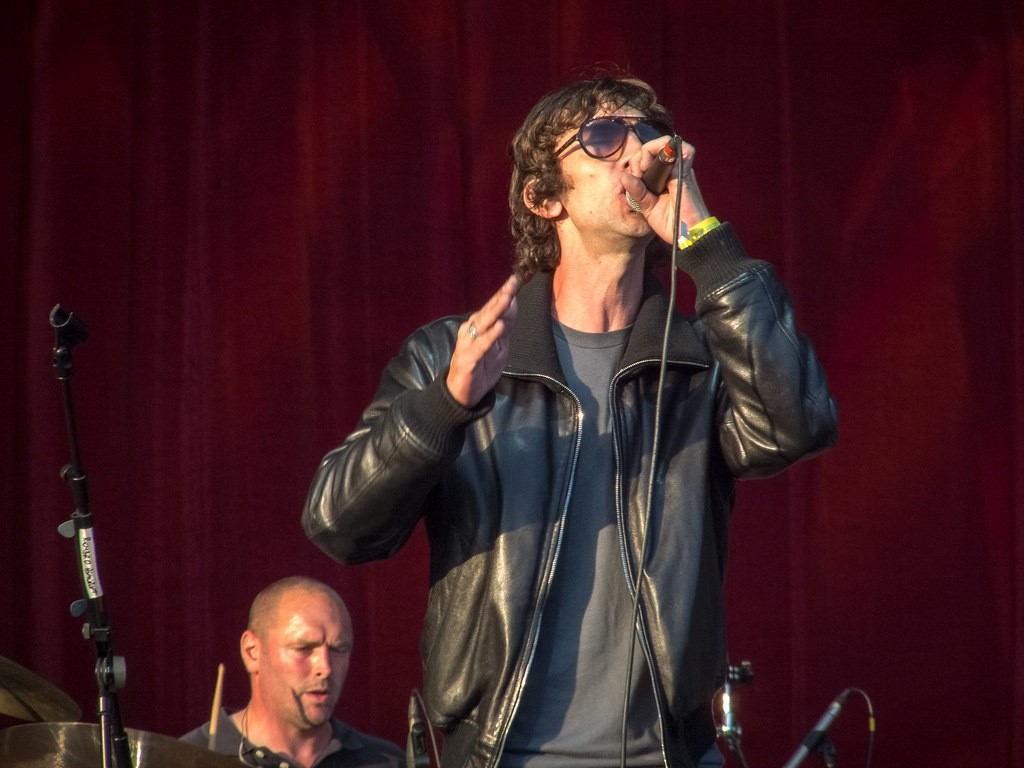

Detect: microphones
left=784, top=688, right=853, bottom=768
left=406, top=695, right=431, bottom=768
left=625, top=136, right=684, bottom=215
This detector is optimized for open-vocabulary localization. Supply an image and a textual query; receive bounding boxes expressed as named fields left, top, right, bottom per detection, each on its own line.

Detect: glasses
left=552, top=116, right=677, bottom=160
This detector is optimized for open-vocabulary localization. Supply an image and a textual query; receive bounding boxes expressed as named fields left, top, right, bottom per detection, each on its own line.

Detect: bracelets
left=677, top=216, right=720, bottom=250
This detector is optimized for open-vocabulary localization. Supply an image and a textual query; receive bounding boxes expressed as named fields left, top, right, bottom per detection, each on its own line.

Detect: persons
left=301, top=75, right=839, bottom=768
left=177, top=575, right=408, bottom=768
